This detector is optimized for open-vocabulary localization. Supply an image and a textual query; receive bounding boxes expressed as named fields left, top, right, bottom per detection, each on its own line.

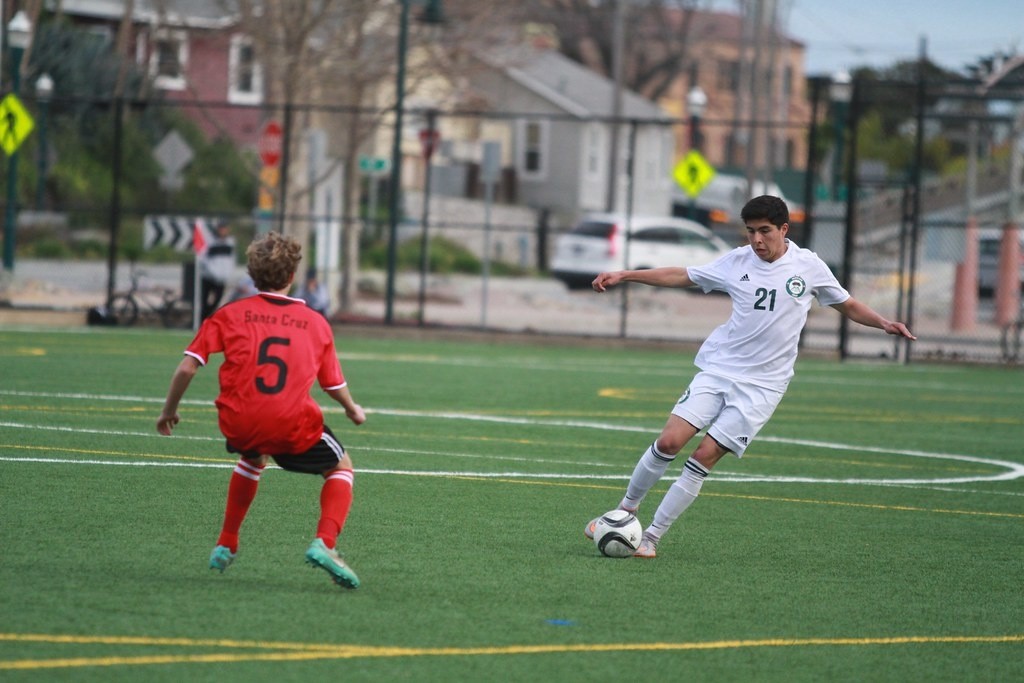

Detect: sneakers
left=304, top=538, right=360, bottom=589
left=632, top=532, right=660, bottom=557
left=584, top=502, right=639, bottom=540
left=209, top=545, right=237, bottom=574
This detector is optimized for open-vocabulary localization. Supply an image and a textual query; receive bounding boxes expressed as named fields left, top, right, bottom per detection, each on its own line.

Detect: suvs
left=550, top=218, right=738, bottom=297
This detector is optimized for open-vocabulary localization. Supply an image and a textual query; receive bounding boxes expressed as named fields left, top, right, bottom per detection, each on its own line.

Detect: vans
left=976, top=230, right=1023, bottom=299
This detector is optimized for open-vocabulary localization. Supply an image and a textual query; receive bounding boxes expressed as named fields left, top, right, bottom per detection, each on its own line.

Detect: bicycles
left=106, top=258, right=195, bottom=329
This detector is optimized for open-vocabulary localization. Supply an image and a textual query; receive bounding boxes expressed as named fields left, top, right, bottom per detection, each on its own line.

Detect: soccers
left=594, top=509, right=642, bottom=558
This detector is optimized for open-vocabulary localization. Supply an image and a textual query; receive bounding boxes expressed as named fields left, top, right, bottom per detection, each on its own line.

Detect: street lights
left=828, top=67, right=851, bottom=205
left=34, top=73, right=54, bottom=212
left=0, top=8, right=34, bottom=272
left=686, top=85, right=707, bottom=223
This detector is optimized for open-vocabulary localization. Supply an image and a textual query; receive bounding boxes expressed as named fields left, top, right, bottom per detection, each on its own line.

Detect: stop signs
left=259, top=123, right=284, bottom=166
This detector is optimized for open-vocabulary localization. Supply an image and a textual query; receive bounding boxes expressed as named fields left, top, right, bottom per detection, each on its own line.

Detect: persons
left=155, top=230, right=367, bottom=589
left=583, top=195, right=917, bottom=558
left=230, top=274, right=258, bottom=302
left=294, top=266, right=328, bottom=313
left=202, top=220, right=235, bottom=314
